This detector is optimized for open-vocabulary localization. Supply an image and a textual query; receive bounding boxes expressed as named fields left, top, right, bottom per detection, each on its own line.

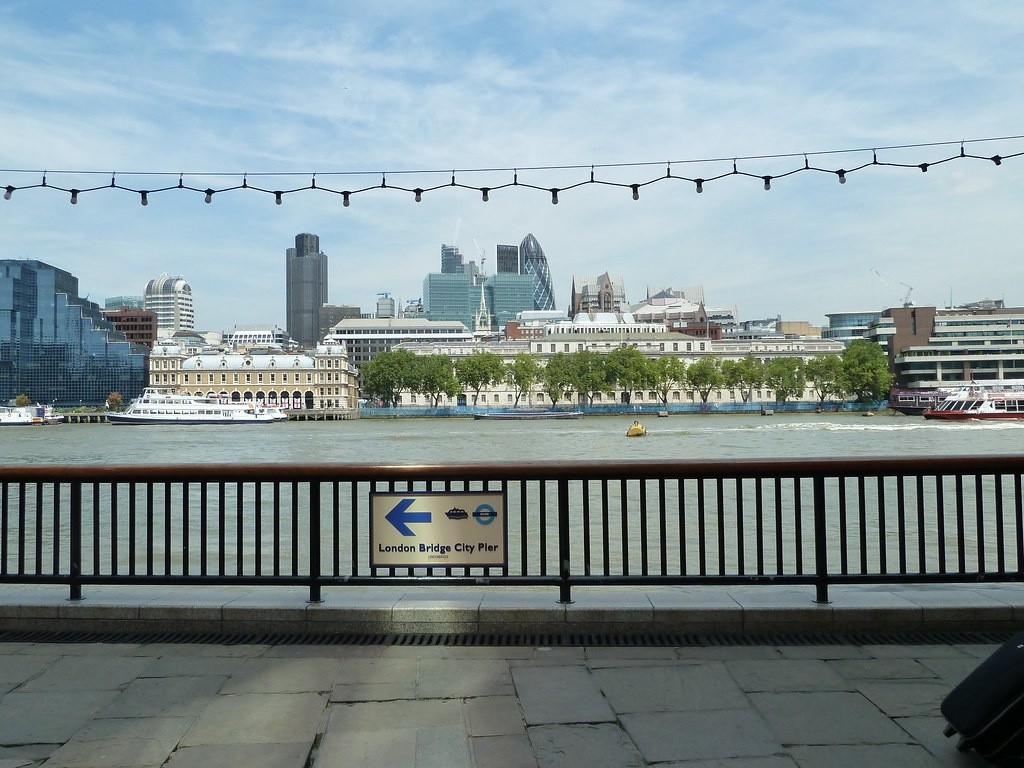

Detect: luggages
left=941, top=630, right=1023, bottom=767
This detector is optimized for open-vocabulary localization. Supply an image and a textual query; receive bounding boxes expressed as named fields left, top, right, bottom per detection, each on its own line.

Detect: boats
left=0, top=403, right=65, bottom=426
left=626, top=421, right=647, bottom=437
left=887, top=386, right=952, bottom=416
left=104, top=387, right=289, bottom=425
left=921, top=381, right=1024, bottom=421
left=474, top=412, right=585, bottom=420
